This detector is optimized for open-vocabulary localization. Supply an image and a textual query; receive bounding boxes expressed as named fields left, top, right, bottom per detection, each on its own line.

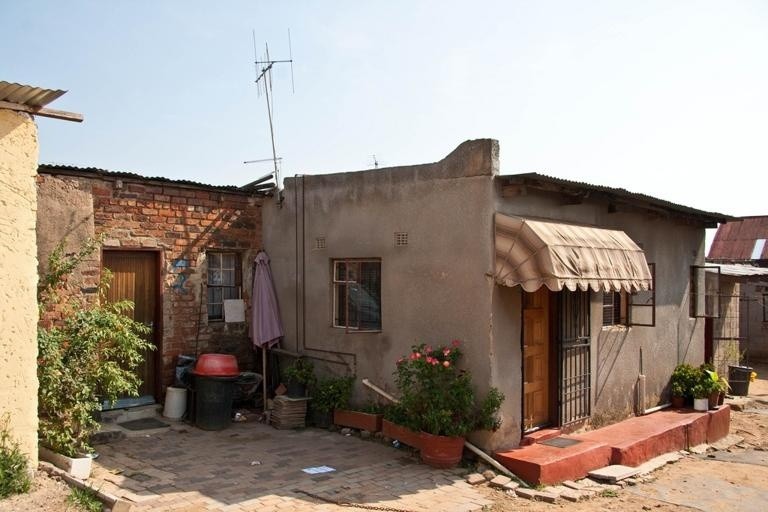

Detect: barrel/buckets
left=194, top=376, right=244, bottom=430
left=727, top=363, right=755, bottom=396
left=162, top=387, right=188, bottom=421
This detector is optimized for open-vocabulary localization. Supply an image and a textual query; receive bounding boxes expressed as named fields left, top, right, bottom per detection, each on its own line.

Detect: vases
left=418, top=429, right=464, bottom=468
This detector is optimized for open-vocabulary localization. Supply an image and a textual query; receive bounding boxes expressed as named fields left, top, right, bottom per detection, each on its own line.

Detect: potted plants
left=282, top=357, right=357, bottom=429
left=332, top=400, right=421, bottom=448
left=667, top=357, right=733, bottom=412
left=38, top=231, right=158, bottom=480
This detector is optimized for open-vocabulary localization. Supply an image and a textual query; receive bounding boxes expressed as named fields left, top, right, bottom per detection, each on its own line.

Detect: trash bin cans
left=728, top=365, right=753, bottom=395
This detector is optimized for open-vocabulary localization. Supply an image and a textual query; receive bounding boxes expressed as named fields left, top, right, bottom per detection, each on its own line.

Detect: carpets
left=117, top=417, right=171, bottom=431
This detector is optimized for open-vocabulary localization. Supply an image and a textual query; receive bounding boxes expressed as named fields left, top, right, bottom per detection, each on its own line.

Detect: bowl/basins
left=233, top=372, right=263, bottom=401
left=191, top=354, right=241, bottom=376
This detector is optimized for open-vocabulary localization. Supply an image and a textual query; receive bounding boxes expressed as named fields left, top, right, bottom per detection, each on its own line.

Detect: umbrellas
left=248, top=252, right=284, bottom=411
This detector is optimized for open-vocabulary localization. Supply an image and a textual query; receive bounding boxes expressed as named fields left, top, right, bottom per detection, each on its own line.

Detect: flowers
left=391, top=335, right=506, bottom=438
